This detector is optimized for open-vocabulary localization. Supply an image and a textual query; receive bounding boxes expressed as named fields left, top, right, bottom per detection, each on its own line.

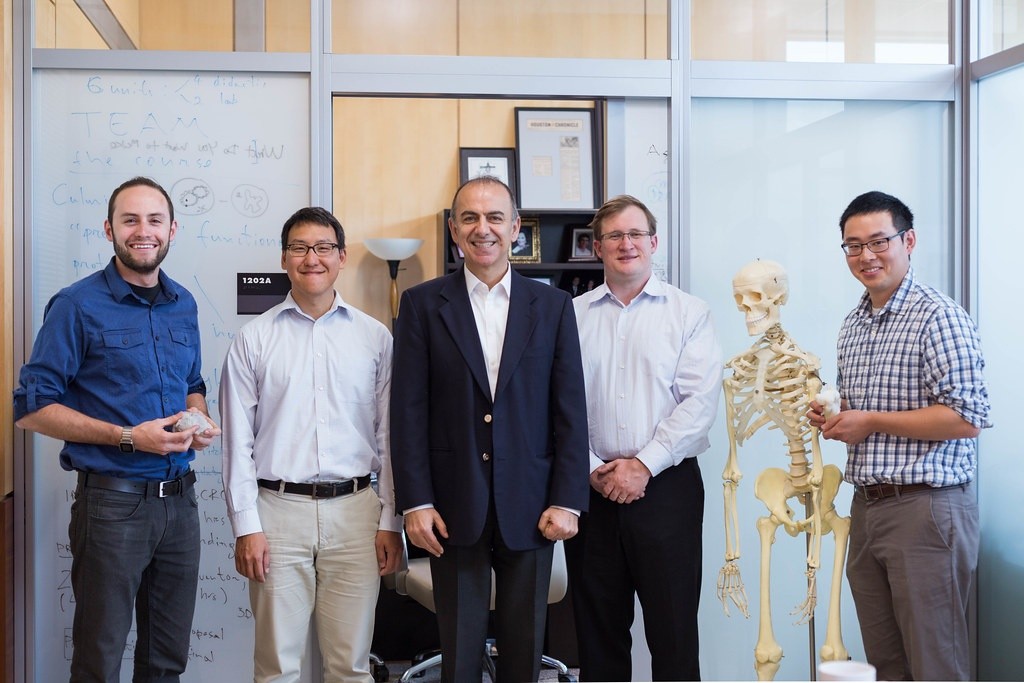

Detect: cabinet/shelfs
left=443, top=208, right=607, bottom=300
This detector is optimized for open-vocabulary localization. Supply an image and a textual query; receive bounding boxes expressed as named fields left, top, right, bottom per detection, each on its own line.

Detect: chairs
left=396, top=534, right=579, bottom=683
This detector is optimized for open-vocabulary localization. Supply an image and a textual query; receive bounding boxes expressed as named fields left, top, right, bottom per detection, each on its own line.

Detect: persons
left=13, top=177, right=222, bottom=683
left=390, top=179, right=587, bottom=683
left=571, top=196, right=710, bottom=683
left=806, top=192, right=990, bottom=682
left=217, top=206, right=404, bottom=683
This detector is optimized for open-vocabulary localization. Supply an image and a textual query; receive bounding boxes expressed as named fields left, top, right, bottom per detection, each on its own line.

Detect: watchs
left=118, top=426, right=135, bottom=453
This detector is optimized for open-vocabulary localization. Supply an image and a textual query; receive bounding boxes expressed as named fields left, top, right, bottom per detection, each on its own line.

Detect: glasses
left=285, top=243, right=339, bottom=257
left=600, top=230, right=650, bottom=240
left=841, top=230, right=907, bottom=256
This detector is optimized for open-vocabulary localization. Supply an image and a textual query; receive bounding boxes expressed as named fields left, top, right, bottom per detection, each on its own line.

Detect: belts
left=77, top=469, right=196, bottom=497
left=258, top=473, right=371, bottom=499
left=853, top=482, right=971, bottom=500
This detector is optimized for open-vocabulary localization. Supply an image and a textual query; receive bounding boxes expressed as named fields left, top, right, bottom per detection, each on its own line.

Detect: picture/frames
left=449, top=233, right=465, bottom=263
left=514, top=105, right=604, bottom=212
left=507, top=217, right=542, bottom=265
left=566, top=222, right=600, bottom=263
left=459, top=146, right=518, bottom=208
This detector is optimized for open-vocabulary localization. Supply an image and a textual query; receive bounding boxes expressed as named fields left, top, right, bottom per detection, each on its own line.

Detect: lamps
left=362, top=236, right=423, bottom=338
